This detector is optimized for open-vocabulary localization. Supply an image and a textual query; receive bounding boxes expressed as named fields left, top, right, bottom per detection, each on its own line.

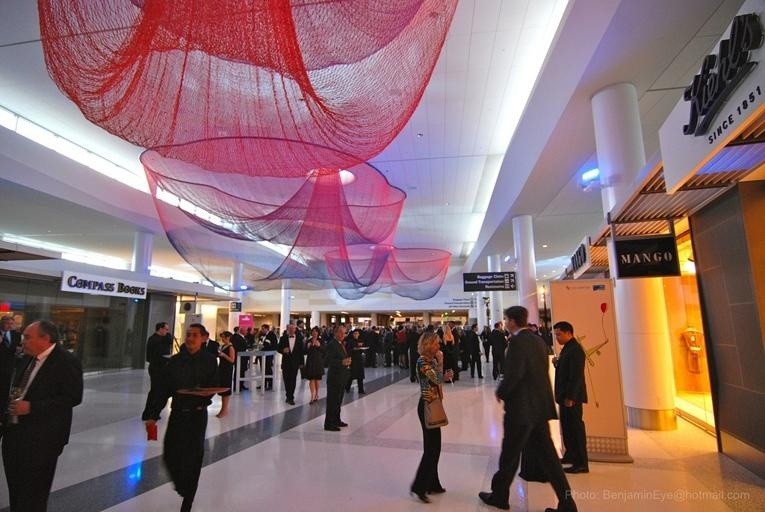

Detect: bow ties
left=290, top=335, right=294, bottom=338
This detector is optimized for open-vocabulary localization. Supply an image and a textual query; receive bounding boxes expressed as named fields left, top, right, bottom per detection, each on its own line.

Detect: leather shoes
left=412, top=457, right=589, bottom=512
left=335, top=422, right=348, bottom=426
left=325, top=425, right=340, bottom=431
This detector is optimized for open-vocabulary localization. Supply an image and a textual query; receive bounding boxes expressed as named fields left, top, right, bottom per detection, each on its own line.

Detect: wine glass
left=347, top=357, right=352, bottom=369
left=446, top=367, right=456, bottom=390
left=217, top=346, right=224, bottom=359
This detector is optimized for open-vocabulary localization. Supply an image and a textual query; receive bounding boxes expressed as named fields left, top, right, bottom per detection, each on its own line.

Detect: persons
left=214, top=331, right=235, bottom=417
left=548, top=319, right=581, bottom=474
left=528, top=323, right=553, bottom=354
left=346, top=324, right=435, bottom=393
left=323, top=325, right=347, bottom=432
left=203, top=332, right=220, bottom=358
left=231, top=320, right=334, bottom=404
left=409, top=332, right=454, bottom=503
left=478, top=306, right=577, bottom=512
left=0, top=302, right=80, bottom=377
left=437, top=321, right=509, bottom=382
left=138, top=324, right=220, bottom=512
left=138, top=322, right=175, bottom=420
left=0, top=318, right=83, bottom=511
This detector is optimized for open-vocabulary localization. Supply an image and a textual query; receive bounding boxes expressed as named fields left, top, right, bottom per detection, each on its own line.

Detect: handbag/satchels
left=424, top=398, right=448, bottom=429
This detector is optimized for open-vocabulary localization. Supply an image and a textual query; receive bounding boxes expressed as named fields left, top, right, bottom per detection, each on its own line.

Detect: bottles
left=8, top=390, right=24, bottom=425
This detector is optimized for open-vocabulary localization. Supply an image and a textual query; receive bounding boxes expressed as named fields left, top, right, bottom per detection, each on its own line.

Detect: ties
left=18, top=356, right=36, bottom=394
left=4, top=333, right=10, bottom=344
